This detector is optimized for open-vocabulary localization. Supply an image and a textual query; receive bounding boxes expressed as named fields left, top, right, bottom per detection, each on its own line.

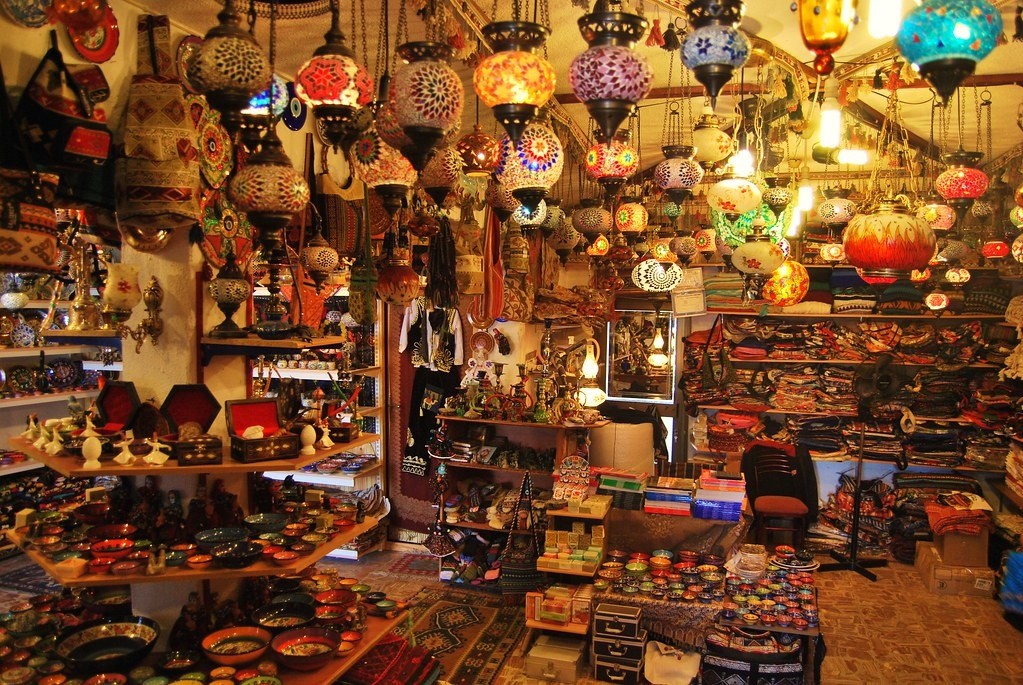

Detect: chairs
left=739, top=441, right=819, bottom=556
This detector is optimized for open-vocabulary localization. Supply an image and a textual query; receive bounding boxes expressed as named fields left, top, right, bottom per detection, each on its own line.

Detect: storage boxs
left=535, top=636, right=587, bottom=653
left=914, top=541, right=994, bottom=597
left=933, top=524, right=988, bottom=567
left=592, top=603, right=643, bottom=639
left=526, top=592, right=544, bottom=620
left=595, top=655, right=645, bottom=685
left=592, top=630, right=648, bottom=661
left=224, top=397, right=299, bottom=464
left=541, top=599, right=571, bottom=627
left=524, top=645, right=583, bottom=684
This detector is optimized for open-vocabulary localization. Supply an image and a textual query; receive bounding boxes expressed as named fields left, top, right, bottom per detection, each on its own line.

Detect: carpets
left=354, top=547, right=529, bottom=685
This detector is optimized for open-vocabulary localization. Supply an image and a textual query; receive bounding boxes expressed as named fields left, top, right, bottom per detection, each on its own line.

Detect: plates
left=176, top=34, right=206, bottom=95
left=466, top=301, right=495, bottom=329
left=469, top=331, right=495, bottom=354
left=67, top=0, right=119, bottom=65
left=2, top=312, right=103, bottom=395
left=0, top=0, right=53, bottom=28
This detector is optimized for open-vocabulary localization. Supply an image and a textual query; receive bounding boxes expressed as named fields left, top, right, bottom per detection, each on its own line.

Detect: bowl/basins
left=593, top=543, right=820, bottom=630
left=0, top=435, right=394, bottom=685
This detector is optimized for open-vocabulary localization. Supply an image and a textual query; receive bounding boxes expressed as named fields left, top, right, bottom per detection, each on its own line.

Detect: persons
left=110, top=475, right=245, bottom=546
left=318, top=425, right=335, bottom=448
left=21, top=415, right=64, bottom=456
left=168, top=575, right=273, bottom=651
left=143, top=438, right=172, bottom=465
left=80, top=415, right=102, bottom=437
left=113, top=436, right=136, bottom=464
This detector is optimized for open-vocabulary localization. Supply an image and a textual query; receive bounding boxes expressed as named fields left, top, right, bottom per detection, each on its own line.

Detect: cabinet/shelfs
left=0, top=86, right=1023, bottom=685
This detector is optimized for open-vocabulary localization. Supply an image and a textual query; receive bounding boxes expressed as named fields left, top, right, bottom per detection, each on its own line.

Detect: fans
left=816, top=349, right=905, bottom=583
